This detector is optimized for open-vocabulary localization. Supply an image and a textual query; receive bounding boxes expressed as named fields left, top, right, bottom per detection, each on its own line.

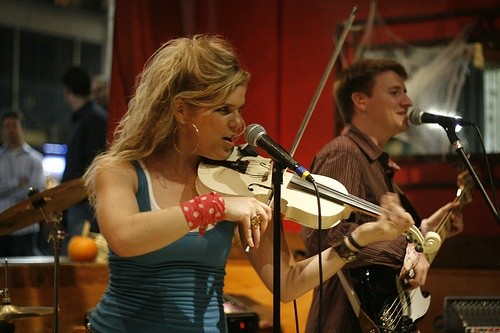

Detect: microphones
left=409, top=109, right=472, bottom=127
left=243, top=123, right=313, bottom=184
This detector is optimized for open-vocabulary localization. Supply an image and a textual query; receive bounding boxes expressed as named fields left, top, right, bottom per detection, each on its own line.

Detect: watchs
left=335, top=240, right=357, bottom=263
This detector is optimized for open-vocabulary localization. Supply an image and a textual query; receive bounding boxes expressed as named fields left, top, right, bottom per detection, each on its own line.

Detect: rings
left=252, top=215, right=261, bottom=228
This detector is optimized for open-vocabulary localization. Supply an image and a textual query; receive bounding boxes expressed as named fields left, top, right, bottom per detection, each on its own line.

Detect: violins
left=193, top=142, right=443, bottom=256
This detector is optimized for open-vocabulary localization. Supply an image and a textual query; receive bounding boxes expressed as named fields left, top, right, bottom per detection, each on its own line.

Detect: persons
left=60, top=64, right=109, bottom=259
left=83, top=35, right=415, bottom=333
left=91, top=74, right=110, bottom=110
left=0, top=110, right=45, bottom=258
left=298, top=58, right=464, bottom=333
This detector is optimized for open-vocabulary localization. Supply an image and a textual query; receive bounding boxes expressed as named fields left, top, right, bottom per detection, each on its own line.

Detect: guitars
left=347, top=166, right=474, bottom=333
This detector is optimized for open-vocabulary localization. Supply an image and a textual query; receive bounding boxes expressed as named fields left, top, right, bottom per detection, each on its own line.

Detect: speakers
left=443, top=295, right=500, bottom=333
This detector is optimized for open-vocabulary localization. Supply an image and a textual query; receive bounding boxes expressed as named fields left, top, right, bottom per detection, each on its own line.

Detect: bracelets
left=349, top=233, right=364, bottom=249
left=180, top=191, right=227, bottom=238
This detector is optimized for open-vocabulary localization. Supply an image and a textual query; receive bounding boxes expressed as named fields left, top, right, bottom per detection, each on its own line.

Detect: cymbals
left=1, top=304, right=62, bottom=322
left=0, top=179, right=90, bottom=229
